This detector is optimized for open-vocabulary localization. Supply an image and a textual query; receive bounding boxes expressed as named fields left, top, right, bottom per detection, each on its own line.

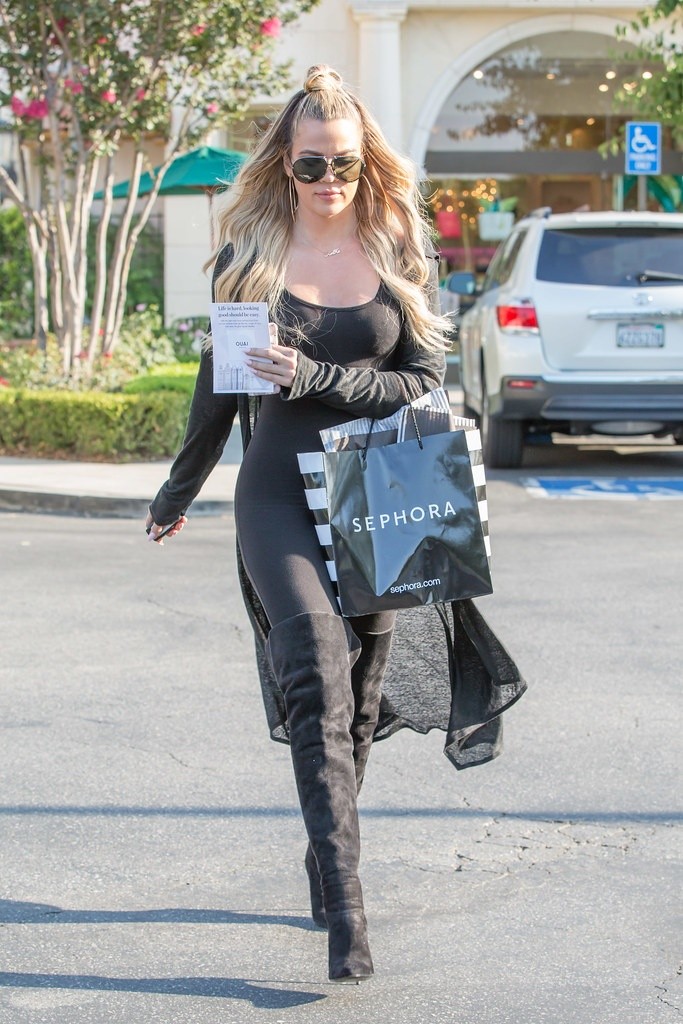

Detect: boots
left=305, top=623, right=395, bottom=928
left=265, top=611, right=374, bottom=983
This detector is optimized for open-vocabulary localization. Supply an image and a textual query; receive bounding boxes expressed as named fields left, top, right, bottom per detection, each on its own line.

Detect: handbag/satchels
left=296, top=385, right=495, bottom=618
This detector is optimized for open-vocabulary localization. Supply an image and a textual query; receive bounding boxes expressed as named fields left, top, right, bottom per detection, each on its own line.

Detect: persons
left=576, top=239, right=615, bottom=285
left=148, top=64, right=458, bottom=985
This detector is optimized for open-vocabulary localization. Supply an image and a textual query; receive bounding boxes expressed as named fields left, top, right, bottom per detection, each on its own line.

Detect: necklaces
left=294, top=217, right=357, bottom=257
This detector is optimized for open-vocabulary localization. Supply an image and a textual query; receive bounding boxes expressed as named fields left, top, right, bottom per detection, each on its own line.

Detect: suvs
left=441, top=207, right=683, bottom=473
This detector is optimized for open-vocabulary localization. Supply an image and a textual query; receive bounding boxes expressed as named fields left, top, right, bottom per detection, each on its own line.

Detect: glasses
left=290, top=153, right=367, bottom=185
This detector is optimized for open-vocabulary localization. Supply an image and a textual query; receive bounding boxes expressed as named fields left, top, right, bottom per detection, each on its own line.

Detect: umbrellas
left=92, top=146, right=256, bottom=271
